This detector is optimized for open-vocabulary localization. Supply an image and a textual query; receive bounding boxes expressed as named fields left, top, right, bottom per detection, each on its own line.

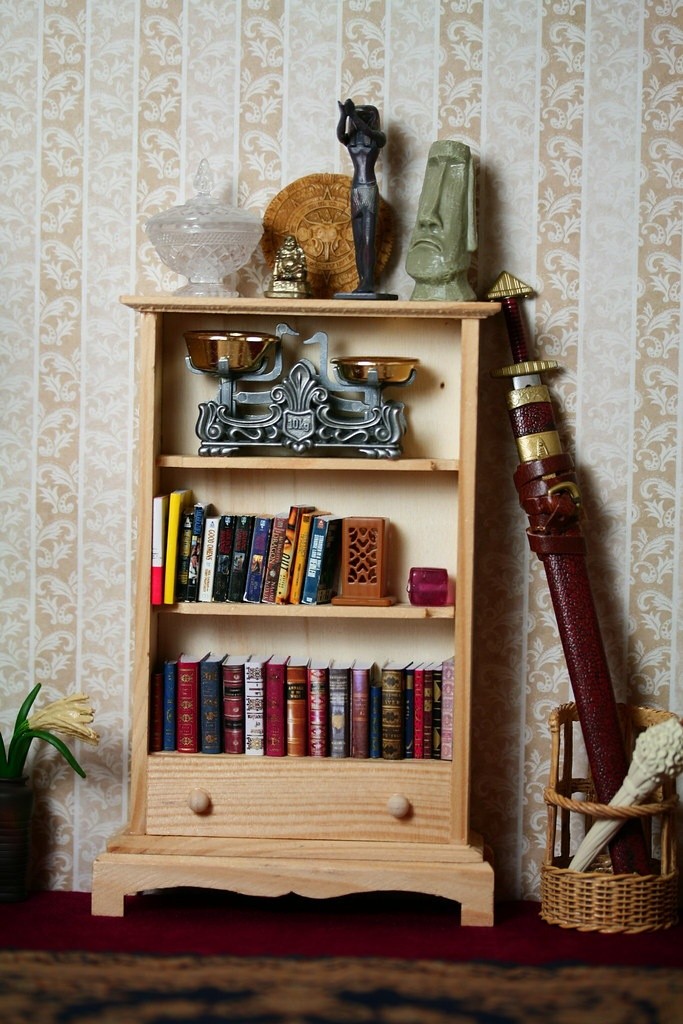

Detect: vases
left=0, top=777, right=33, bottom=902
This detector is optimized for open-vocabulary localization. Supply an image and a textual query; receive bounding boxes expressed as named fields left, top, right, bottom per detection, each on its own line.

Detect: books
left=149, top=485, right=455, bottom=761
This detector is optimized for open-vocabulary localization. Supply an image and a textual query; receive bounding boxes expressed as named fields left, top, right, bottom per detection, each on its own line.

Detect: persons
left=335, top=98, right=387, bottom=294
left=405, top=140, right=480, bottom=301
left=272, top=236, right=307, bottom=282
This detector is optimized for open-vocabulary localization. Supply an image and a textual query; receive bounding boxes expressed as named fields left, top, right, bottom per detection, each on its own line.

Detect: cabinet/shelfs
left=90, top=293, right=502, bottom=925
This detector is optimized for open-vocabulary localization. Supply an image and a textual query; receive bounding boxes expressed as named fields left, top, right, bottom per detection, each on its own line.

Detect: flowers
left=0, top=683, right=101, bottom=776
